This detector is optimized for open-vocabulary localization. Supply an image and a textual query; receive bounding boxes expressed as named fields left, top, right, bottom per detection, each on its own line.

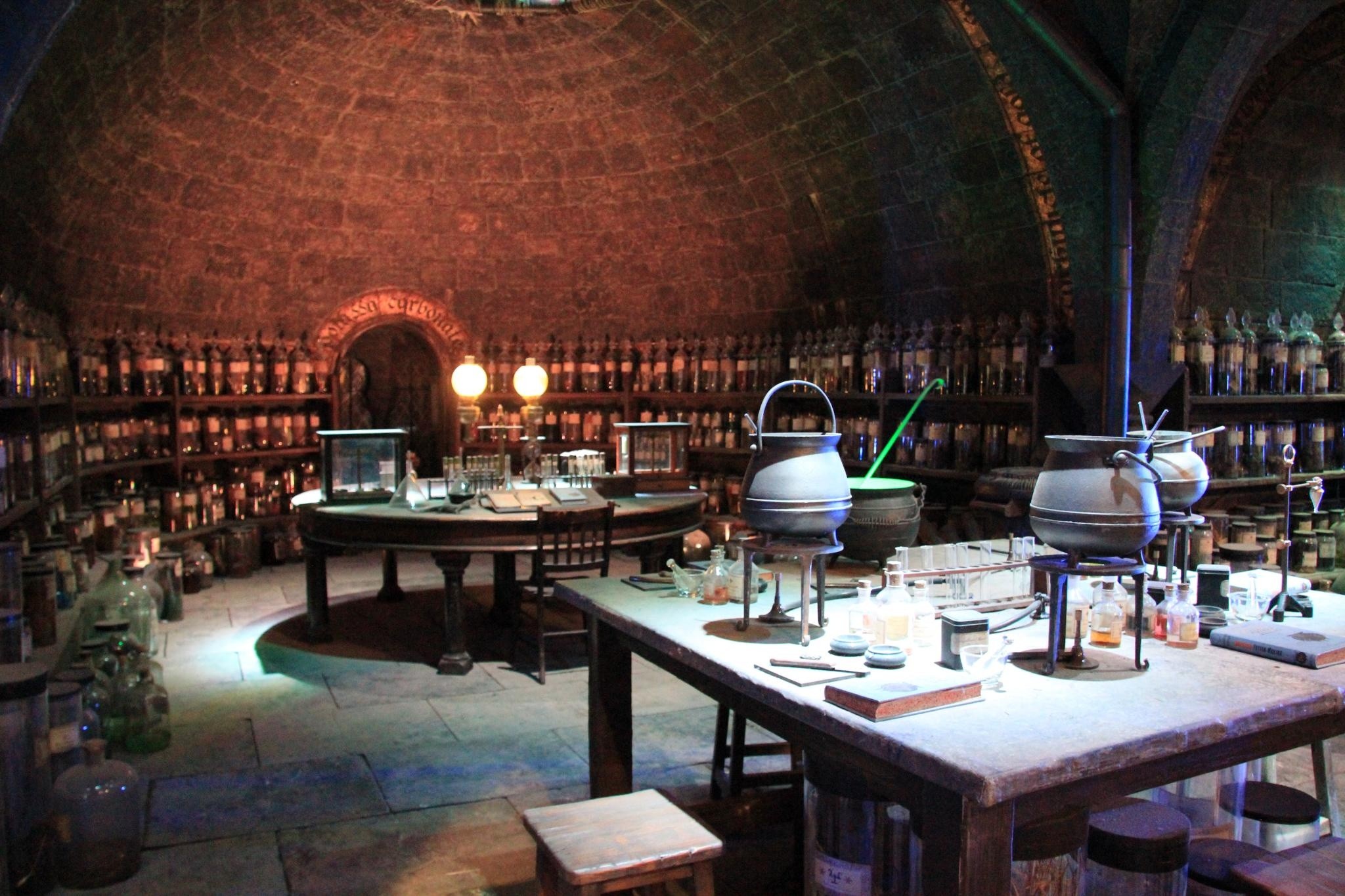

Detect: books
left=549, top=488, right=588, bottom=506
left=824, top=664, right=985, bottom=722
left=1210, top=620, right=1345, bottom=669
left=688, top=559, right=774, bottom=583
left=487, top=493, right=551, bottom=510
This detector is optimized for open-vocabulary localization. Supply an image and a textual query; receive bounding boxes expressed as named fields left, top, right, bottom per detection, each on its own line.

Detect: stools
left=709, top=704, right=805, bottom=802
left=523, top=787, right=725, bottom=895
left=1230, top=835, right=1345, bottom=894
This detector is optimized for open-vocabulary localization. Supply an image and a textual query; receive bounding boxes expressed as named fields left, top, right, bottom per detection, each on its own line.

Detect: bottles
left=1189, top=414, right=1345, bottom=478
left=1168, top=306, right=1345, bottom=397
left=0, top=664, right=53, bottom=894
left=846, top=561, right=937, bottom=659
left=1065, top=573, right=1200, bottom=648
left=802, top=743, right=1324, bottom=896
left=464, top=311, right=1052, bottom=512
left=1147, top=500, right=1345, bottom=575
left=48, top=739, right=140, bottom=892
left=702, top=545, right=760, bottom=604
left=0, top=291, right=328, bottom=771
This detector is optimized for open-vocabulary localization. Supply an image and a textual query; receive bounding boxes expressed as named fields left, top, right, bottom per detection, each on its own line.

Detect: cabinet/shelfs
left=0, top=347, right=1344, bottom=702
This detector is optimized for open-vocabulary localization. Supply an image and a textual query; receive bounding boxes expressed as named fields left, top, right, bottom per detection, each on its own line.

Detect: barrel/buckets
left=835, top=478, right=928, bottom=569
left=1124, top=428, right=1209, bottom=516
left=737, top=379, right=853, bottom=544
left=1027, top=435, right=1163, bottom=558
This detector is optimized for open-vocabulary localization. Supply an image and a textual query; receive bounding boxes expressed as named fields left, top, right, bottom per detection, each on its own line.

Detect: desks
left=288, top=488, right=710, bottom=672
left=554, top=539, right=1344, bottom=895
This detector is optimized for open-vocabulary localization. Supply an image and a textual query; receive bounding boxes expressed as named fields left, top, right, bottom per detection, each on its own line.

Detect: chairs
left=507, top=499, right=616, bottom=686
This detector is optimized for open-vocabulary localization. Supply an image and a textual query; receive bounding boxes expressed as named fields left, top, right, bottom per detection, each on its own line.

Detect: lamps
left=513, top=357, right=549, bottom=481
left=450, top=356, right=487, bottom=488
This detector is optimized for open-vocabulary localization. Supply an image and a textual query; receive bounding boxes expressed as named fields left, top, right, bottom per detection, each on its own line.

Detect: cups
left=1192, top=604, right=1228, bottom=635
left=1227, top=592, right=1271, bottom=623
left=960, top=645, right=1006, bottom=688
left=672, top=569, right=704, bottom=597
